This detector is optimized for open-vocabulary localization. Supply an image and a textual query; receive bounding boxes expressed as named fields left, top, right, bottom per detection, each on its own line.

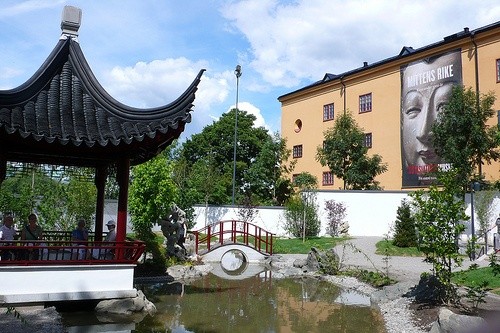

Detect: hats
left=106, top=220, right=116, bottom=226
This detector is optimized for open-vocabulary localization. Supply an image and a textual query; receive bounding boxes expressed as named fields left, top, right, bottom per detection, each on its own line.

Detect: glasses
left=107, top=225, right=112, bottom=227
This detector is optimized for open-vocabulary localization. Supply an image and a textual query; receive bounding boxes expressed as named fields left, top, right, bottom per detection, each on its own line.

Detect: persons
left=22, top=214, right=48, bottom=259
left=72, top=220, right=88, bottom=260
left=0, top=216, right=18, bottom=261
left=89, top=220, right=116, bottom=260
left=161, top=202, right=189, bottom=256
